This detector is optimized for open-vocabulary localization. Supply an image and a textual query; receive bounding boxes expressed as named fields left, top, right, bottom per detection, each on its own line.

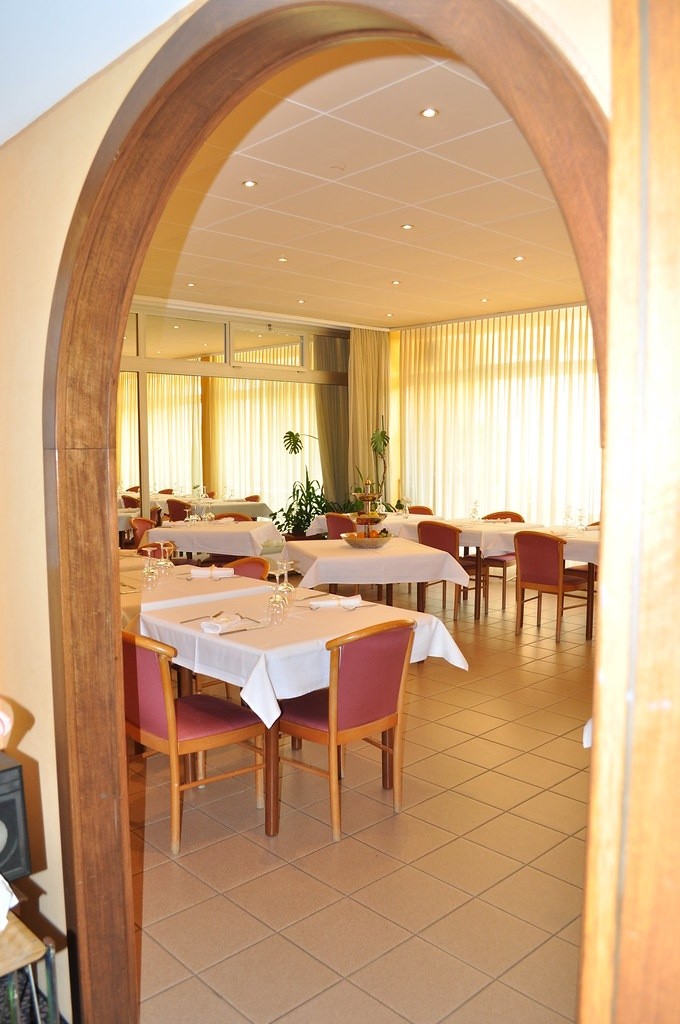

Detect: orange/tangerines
left=357, top=533, right=365, bottom=538
left=370, top=530, right=379, bottom=538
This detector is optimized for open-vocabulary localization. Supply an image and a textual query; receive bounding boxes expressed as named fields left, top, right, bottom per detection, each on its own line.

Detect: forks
left=180, top=611, right=224, bottom=624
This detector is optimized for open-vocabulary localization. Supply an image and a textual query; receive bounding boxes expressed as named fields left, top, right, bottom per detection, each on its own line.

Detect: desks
left=117, top=494, right=575, bottom=838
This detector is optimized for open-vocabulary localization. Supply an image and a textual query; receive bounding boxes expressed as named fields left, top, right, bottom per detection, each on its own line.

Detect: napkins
left=309, top=600, right=338, bottom=610
left=340, top=598, right=362, bottom=607
left=222, top=516, right=234, bottom=521
left=213, top=520, right=225, bottom=524
left=192, top=568, right=234, bottom=579
left=200, top=612, right=242, bottom=634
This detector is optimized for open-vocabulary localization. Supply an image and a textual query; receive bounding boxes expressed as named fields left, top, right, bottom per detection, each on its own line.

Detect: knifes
left=219, top=625, right=264, bottom=635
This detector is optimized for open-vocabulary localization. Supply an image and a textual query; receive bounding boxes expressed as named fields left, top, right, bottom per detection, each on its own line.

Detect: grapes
left=379, top=528, right=391, bottom=538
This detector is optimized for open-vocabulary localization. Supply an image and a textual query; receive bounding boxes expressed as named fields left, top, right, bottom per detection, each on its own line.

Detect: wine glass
left=221, top=486, right=234, bottom=503
left=273, top=560, right=296, bottom=609
left=267, top=570, right=289, bottom=621
left=266, top=585, right=282, bottom=625
left=201, top=485, right=209, bottom=498
left=142, top=540, right=174, bottom=591
left=183, top=501, right=215, bottom=528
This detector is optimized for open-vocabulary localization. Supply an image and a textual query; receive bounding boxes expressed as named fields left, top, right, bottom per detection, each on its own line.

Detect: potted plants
left=317, top=487, right=364, bottom=538
left=271, top=432, right=326, bottom=539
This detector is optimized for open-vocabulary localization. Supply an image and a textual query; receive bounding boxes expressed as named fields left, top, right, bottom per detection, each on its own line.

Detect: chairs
left=245, top=494, right=261, bottom=521
left=200, top=511, right=253, bottom=566
left=514, top=530, right=595, bottom=643
left=192, top=556, right=270, bottom=695
left=166, top=498, right=194, bottom=522
left=253, top=617, right=417, bottom=844
left=129, top=517, right=156, bottom=547
left=396, top=506, right=435, bottom=595
left=325, top=512, right=384, bottom=600
left=120, top=629, right=266, bottom=854
left=464, top=511, right=524, bottom=609
left=137, top=540, right=174, bottom=558
left=120, top=494, right=140, bottom=508
left=416, top=520, right=490, bottom=620
